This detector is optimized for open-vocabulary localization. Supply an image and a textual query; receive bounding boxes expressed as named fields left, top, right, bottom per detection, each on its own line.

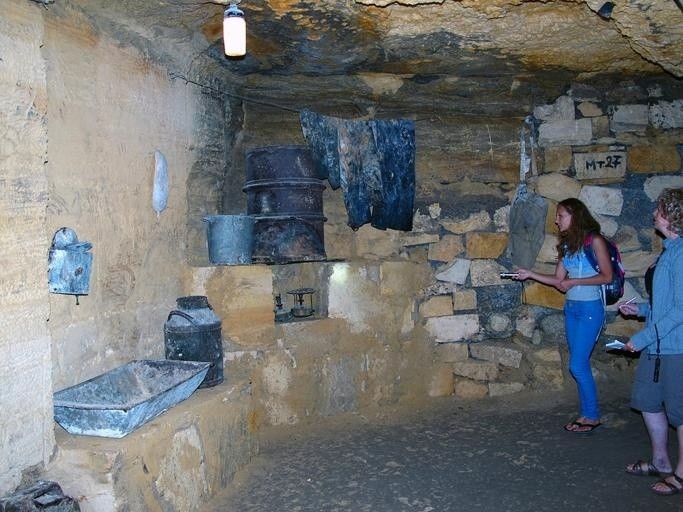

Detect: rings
left=631, top=350, right=635, bottom=353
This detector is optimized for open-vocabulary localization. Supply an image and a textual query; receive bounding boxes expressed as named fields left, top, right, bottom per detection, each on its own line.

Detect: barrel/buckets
left=243, top=147, right=329, bottom=265
left=200, top=213, right=258, bottom=265
left=163, top=295, right=225, bottom=390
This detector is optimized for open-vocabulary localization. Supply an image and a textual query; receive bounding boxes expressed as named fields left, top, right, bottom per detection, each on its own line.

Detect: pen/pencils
left=625, top=296, right=637, bottom=306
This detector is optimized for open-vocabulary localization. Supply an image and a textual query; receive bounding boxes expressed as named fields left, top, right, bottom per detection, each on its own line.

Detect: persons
left=617, top=187, right=683, bottom=496
left=511, top=198, right=613, bottom=434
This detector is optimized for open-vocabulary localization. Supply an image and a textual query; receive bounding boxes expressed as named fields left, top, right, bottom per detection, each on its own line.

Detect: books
left=606, top=339, right=626, bottom=349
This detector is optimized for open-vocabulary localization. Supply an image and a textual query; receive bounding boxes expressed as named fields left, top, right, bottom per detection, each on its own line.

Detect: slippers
left=565, top=418, right=600, bottom=432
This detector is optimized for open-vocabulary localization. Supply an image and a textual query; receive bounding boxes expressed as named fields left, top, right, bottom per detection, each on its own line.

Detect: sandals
left=650, top=471, right=682, bottom=495
left=624, top=459, right=670, bottom=477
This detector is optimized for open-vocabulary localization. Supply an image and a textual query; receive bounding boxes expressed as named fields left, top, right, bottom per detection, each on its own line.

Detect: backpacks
left=585, top=232, right=625, bottom=305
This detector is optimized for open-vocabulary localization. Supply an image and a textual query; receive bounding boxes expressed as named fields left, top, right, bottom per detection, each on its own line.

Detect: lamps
left=222, top=2, right=247, bottom=59
